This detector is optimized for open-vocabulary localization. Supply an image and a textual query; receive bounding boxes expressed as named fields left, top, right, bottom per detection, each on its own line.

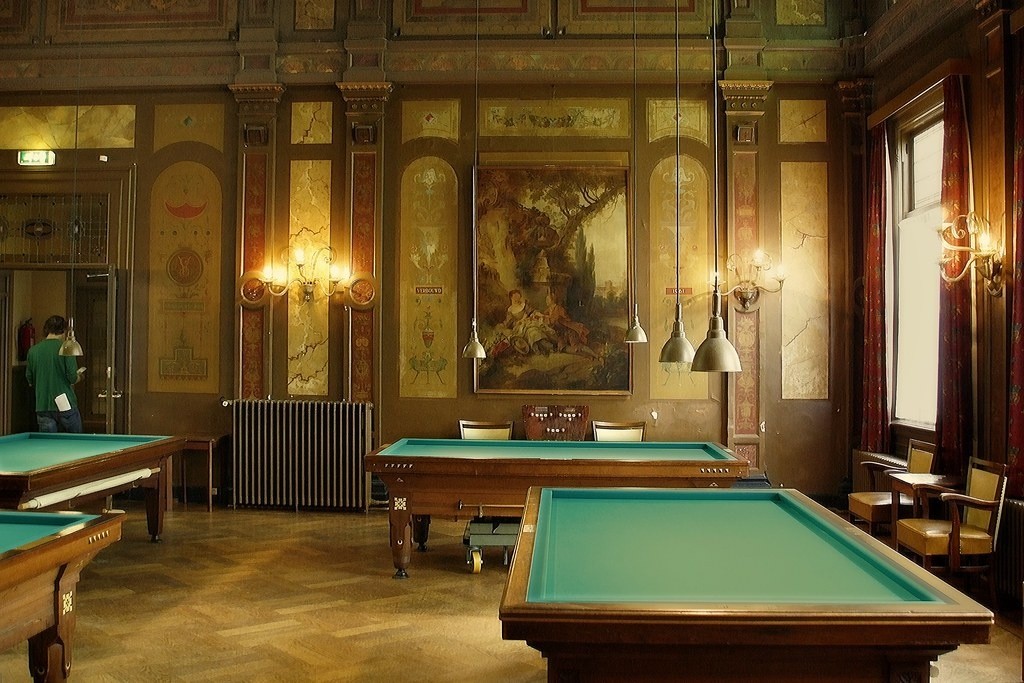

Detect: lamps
left=901, top=207, right=1002, bottom=293
left=618, top=0, right=649, bottom=343
left=660, top=0, right=698, bottom=364
left=714, top=247, right=791, bottom=311
left=253, top=243, right=345, bottom=302
left=691, top=0, right=742, bottom=373
left=460, top=0, right=489, bottom=360
left=58, top=0, right=85, bottom=357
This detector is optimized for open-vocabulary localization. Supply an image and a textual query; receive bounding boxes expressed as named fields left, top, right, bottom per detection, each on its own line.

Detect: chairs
left=849, top=438, right=938, bottom=535
left=901, top=456, right=1010, bottom=596
left=459, top=420, right=515, bottom=443
left=591, top=419, right=647, bottom=441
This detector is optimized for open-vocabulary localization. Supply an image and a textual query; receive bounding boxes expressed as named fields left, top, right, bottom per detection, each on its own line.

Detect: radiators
left=229, top=398, right=371, bottom=514
left=853, top=449, right=907, bottom=493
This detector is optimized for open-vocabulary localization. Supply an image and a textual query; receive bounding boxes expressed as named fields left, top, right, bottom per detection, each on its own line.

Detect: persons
left=25, top=315, right=82, bottom=433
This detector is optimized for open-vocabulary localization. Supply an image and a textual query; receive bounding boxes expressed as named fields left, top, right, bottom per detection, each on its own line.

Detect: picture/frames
left=471, top=163, right=633, bottom=397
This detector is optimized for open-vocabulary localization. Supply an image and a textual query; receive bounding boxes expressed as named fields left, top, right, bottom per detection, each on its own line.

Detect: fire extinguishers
left=17, top=318, right=36, bottom=362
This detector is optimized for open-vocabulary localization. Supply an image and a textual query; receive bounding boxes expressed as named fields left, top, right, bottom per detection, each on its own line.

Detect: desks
left=0, top=509, right=128, bottom=683
left=360, top=434, right=750, bottom=578
left=892, top=473, right=956, bottom=549
left=500, top=485, right=993, bottom=683
left=166, top=436, right=225, bottom=509
left=0, top=432, right=186, bottom=542
